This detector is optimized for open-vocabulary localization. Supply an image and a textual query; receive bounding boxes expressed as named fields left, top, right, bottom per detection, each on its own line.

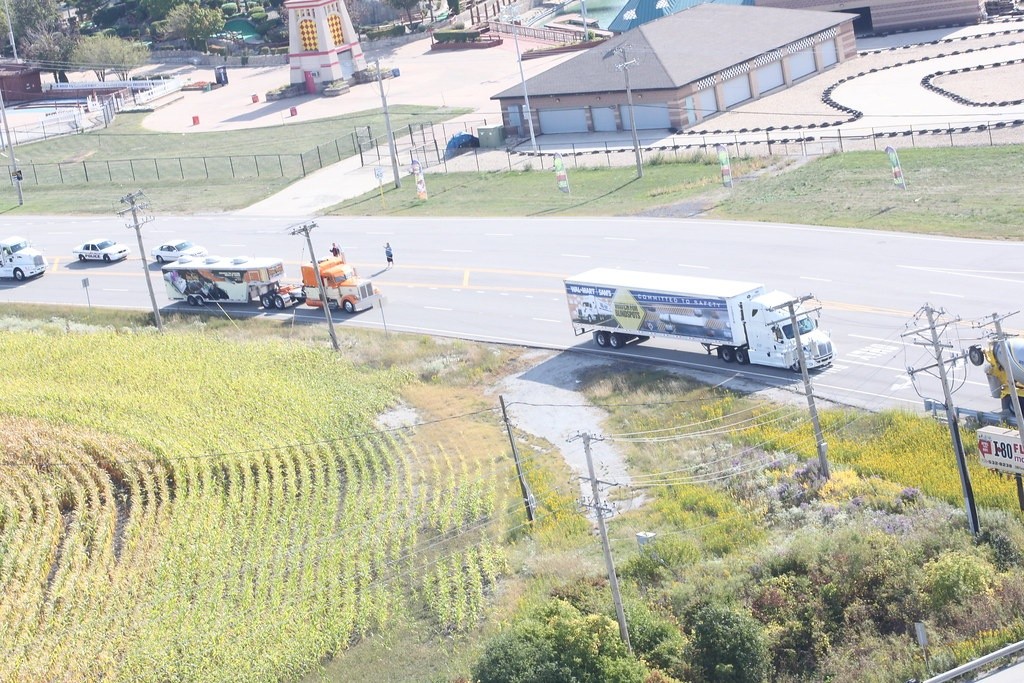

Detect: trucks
left=986, top=334, right=1024, bottom=417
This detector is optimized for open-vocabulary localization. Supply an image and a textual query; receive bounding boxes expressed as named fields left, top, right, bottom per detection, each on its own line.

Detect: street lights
left=499, top=4, right=538, bottom=156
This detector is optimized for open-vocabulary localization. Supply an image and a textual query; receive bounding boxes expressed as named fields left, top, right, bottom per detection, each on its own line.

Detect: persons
left=329, top=243, right=341, bottom=258
left=383, top=243, right=394, bottom=267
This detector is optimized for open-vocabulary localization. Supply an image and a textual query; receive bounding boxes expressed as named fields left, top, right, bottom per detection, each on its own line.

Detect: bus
left=0, top=237, right=48, bottom=281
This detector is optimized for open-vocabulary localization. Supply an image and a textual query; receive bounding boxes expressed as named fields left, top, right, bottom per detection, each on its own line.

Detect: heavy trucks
left=161, top=255, right=379, bottom=314
left=564, top=268, right=837, bottom=373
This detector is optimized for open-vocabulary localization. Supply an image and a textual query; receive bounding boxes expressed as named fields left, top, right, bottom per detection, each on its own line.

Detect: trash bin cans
left=392, top=68, right=400, bottom=77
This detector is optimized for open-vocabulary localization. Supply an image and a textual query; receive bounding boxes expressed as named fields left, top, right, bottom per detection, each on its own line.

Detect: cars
left=73, top=239, right=128, bottom=262
left=150, top=240, right=208, bottom=263
